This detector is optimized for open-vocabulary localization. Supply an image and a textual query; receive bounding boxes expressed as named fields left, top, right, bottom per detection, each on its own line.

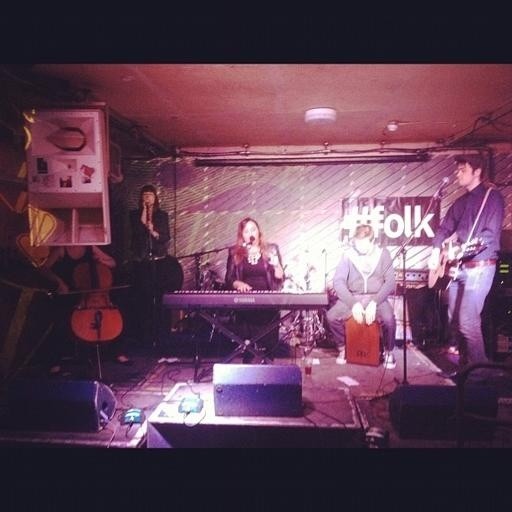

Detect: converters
left=120, top=409, right=144, bottom=425
left=178, top=397, right=203, bottom=413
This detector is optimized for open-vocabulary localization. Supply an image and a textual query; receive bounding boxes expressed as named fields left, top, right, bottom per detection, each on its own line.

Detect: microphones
left=247, top=236, right=255, bottom=248
left=432, top=177, right=449, bottom=198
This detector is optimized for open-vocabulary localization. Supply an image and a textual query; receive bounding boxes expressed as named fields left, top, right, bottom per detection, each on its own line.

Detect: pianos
left=161, top=288, right=329, bottom=310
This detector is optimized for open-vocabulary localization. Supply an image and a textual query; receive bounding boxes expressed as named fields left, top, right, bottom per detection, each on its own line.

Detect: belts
left=457, top=258, right=498, bottom=268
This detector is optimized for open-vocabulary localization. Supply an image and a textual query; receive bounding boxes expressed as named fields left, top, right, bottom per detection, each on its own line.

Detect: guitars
left=428, top=237, right=489, bottom=290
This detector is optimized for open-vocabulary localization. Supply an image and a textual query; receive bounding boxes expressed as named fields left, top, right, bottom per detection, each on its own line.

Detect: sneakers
left=382, top=346, right=396, bottom=369
left=336, top=347, right=346, bottom=365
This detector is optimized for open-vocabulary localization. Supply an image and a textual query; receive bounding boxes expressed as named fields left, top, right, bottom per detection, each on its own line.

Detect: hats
left=454, top=153, right=485, bottom=163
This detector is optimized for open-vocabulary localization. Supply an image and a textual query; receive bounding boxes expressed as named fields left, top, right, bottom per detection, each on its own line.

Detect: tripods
left=368, top=249, right=408, bottom=403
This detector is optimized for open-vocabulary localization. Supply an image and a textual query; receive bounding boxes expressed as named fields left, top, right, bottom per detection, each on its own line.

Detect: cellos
left=71, top=242, right=125, bottom=343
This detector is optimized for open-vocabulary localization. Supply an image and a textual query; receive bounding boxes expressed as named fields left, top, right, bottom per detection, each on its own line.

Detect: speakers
left=388, top=385, right=498, bottom=440
left=39, top=378, right=117, bottom=433
left=213, top=364, right=303, bottom=417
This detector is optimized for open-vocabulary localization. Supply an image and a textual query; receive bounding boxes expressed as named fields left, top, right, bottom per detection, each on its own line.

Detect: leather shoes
left=437, top=364, right=463, bottom=378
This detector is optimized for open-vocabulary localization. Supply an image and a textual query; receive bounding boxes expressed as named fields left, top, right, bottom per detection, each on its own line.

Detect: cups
left=305, top=356, right=313, bottom=375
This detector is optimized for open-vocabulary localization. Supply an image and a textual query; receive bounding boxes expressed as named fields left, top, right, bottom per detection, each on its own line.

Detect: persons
left=116, top=183, right=180, bottom=366
left=326, top=224, right=398, bottom=371
left=427, top=153, right=505, bottom=379
left=223, top=217, right=285, bottom=349
